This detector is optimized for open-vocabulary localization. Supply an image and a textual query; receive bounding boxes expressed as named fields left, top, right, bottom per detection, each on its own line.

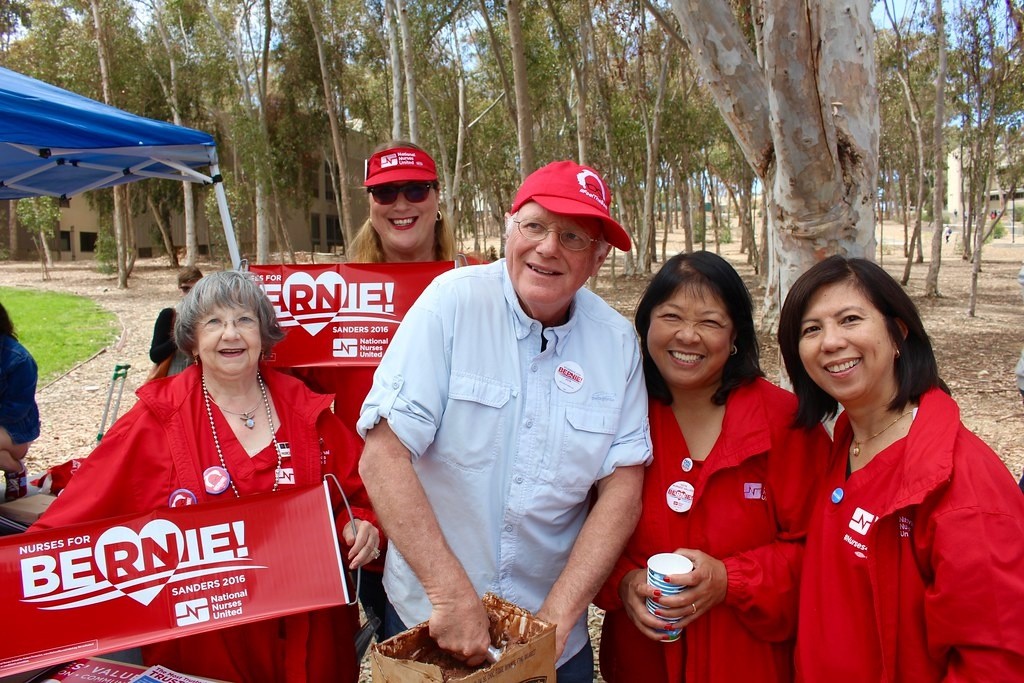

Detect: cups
left=647, top=552, right=694, bottom=643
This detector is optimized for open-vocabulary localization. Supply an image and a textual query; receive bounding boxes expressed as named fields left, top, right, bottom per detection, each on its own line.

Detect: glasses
left=512, top=218, right=604, bottom=251
left=367, top=180, right=436, bottom=205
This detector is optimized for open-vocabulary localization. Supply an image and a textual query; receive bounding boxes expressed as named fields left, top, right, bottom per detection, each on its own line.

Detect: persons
left=316, top=139, right=490, bottom=440
left=944, top=226, right=952, bottom=242
left=0, top=301, right=40, bottom=502
left=150, top=266, right=203, bottom=376
left=776, top=254, right=1024, bottom=682
left=25, top=268, right=385, bottom=683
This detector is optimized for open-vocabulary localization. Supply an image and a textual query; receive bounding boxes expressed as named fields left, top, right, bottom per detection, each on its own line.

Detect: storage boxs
left=0, top=494, right=57, bottom=527
left=370, top=593, right=556, bottom=683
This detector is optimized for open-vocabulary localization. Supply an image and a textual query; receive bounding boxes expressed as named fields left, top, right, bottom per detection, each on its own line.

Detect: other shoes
left=3, top=460, right=29, bottom=501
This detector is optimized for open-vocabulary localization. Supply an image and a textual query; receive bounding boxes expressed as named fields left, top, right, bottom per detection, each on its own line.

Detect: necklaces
left=206, top=395, right=264, bottom=429
left=201, top=371, right=281, bottom=499
left=590, top=250, right=832, bottom=681
left=852, top=410, right=912, bottom=456
left=692, top=604, right=696, bottom=614
left=356, top=160, right=655, bottom=683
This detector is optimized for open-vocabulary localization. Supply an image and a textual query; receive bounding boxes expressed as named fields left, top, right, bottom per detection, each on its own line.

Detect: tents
left=0, top=63, right=244, bottom=271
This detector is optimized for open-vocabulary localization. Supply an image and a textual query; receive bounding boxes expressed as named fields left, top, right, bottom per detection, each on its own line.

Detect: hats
left=362, top=145, right=439, bottom=187
left=510, top=160, right=632, bottom=252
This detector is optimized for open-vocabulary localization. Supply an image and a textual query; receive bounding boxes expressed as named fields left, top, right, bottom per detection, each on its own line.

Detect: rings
left=373, top=547, right=381, bottom=560
